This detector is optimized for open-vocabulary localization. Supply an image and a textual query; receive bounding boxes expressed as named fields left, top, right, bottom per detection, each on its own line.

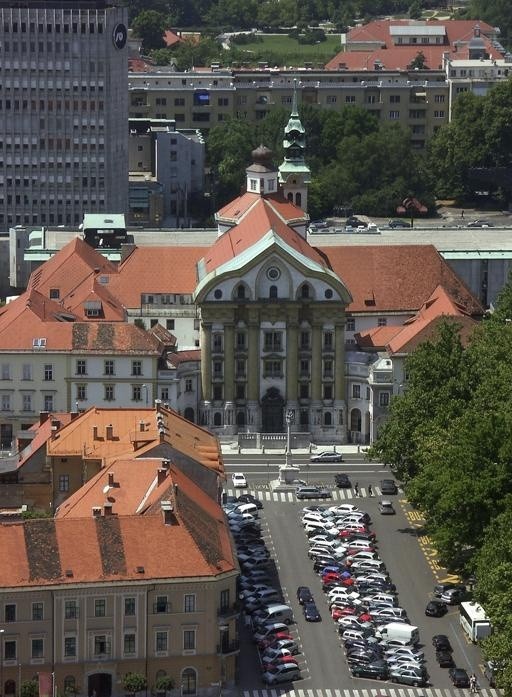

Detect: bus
left=461, top=601, right=492, bottom=643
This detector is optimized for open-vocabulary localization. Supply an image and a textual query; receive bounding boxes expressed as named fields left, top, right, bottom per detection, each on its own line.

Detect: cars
left=221, top=494, right=301, bottom=686
left=426, top=600, right=448, bottom=618
left=485, top=659, right=508, bottom=687
left=335, top=473, right=351, bottom=487
left=433, top=580, right=468, bottom=604
left=296, top=503, right=432, bottom=686
left=379, top=477, right=398, bottom=496
left=377, top=500, right=395, bottom=515
left=432, top=633, right=470, bottom=688
left=309, top=451, right=343, bottom=464
left=230, top=471, right=247, bottom=487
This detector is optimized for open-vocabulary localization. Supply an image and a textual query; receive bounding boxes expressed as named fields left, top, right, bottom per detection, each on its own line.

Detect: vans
left=296, top=484, right=332, bottom=499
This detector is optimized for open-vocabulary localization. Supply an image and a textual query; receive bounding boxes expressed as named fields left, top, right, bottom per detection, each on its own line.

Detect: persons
left=368, top=484, right=372, bottom=497
left=470, top=676, right=475, bottom=694
left=472, top=673, right=477, bottom=687
left=355, top=482, right=359, bottom=496
